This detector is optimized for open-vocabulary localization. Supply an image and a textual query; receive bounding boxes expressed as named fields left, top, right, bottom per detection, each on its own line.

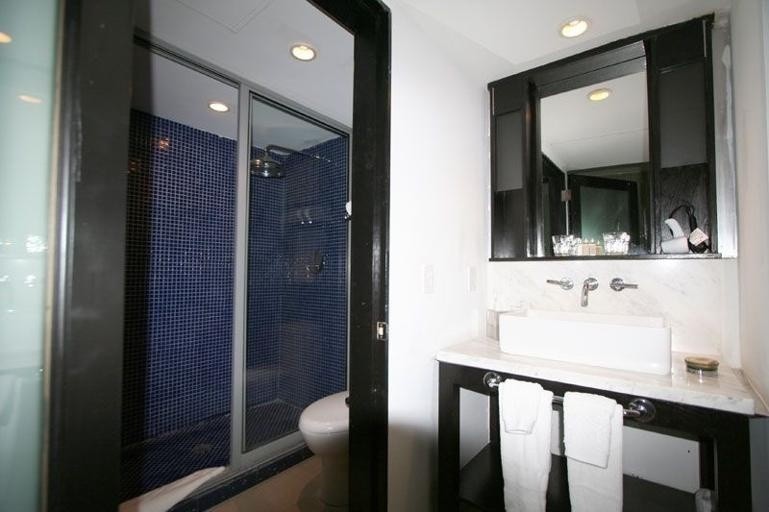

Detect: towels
left=497, top=377, right=626, bottom=512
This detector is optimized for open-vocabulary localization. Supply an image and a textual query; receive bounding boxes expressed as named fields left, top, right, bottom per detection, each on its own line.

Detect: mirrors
left=529, top=54, right=654, bottom=257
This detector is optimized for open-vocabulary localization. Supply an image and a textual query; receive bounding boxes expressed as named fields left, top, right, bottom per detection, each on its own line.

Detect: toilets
left=298, top=392, right=349, bottom=512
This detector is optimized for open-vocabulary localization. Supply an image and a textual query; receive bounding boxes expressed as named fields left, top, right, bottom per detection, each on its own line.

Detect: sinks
left=498, top=309, right=673, bottom=376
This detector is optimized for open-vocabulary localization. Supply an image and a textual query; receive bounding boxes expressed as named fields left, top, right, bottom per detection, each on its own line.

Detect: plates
left=684, top=357, right=720, bottom=371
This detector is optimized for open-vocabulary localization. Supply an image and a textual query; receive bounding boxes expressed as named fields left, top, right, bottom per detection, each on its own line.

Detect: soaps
left=684, top=356, right=721, bottom=372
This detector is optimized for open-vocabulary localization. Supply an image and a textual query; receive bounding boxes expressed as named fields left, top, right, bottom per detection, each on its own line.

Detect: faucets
left=579, top=278, right=598, bottom=306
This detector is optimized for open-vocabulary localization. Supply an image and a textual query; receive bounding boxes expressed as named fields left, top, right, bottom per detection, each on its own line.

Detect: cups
left=550, top=233, right=631, bottom=258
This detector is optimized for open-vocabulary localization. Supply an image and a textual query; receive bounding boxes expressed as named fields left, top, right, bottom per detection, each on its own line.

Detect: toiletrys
left=589, top=238, right=596, bottom=256
left=696, top=488, right=716, bottom=508
left=582, top=238, right=589, bottom=255
left=594, top=240, right=603, bottom=256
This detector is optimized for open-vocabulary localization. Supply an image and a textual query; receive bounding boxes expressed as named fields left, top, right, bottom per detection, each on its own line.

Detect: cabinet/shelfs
left=436, top=361, right=754, bottom=512
